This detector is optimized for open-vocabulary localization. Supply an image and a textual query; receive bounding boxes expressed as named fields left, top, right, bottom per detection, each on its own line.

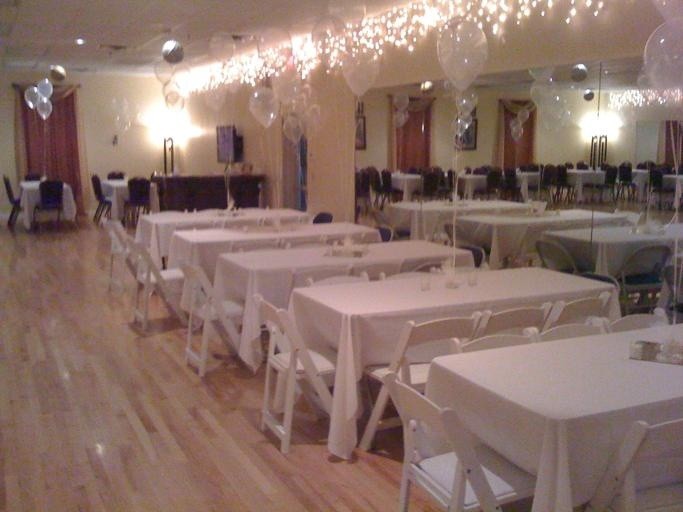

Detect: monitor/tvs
left=216, top=124, right=244, bottom=164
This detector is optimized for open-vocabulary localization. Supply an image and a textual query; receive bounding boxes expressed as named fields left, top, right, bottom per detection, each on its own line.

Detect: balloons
left=644, top=18, right=683, bottom=103
left=393, top=91, right=410, bottom=111
left=637, top=65, right=665, bottom=102
left=209, top=30, right=235, bottom=65
left=537, top=94, right=564, bottom=133
left=257, top=25, right=292, bottom=70
left=393, top=112, right=405, bottom=129
left=273, top=77, right=297, bottom=106
left=227, top=74, right=242, bottom=95
left=283, top=117, right=303, bottom=144
left=292, top=84, right=316, bottom=115
left=249, top=87, right=280, bottom=129
left=528, top=51, right=557, bottom=81
left=165, top=95, right=185, bottom=115
left=112, top=94, right=139, bottom=136
left=37, top=98, right=53, bottom=120
left=517, top=109, right=530, bottom=126
left=300, top=115, right=317, bottom=141
left=311, top=16, right=348, bottom=68
left=202, top=80, right=226, bottom=112
left=443, top=81, right=478, bottom=138
left=155, top=61, right=173, bottom=84
left=511, top=127, right=524, bottom=142
left=37, top=78, right=53, bottom=99
left=309, top=105, right=326, bottom=130
left=653, top=0, right=683, bottom=26
left=530, top=78, right=559, bottom=107
left=342, top=45, right=379, bottom=97
left=510, top=118, right=521, bottom=130
left=24, top=86, right=40, bottom=109
left=173, top=64, right=191, bottom=89
left=436, top=16, right=489, bottom=92
left=162, top=80, right=180, bottom=105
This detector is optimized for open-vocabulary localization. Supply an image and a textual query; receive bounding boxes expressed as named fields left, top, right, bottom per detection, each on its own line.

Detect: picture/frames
left=452, top=118, right=478, bottom=150
left=354, top=116, right=367, bottom=151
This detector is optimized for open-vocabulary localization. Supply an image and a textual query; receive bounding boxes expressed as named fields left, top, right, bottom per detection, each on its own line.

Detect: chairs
left=173, top=255, right=244, bottom=377
left=459, top=334, right=532, bottom=353
left=383, top=372, right=536, bottom=512
left=3, top=174, right=24, bottom=229
left=472, top=301, right=554, bottom=338
left=126, top=239, right=189, bottom=334
left=356, top=311, right=478, bottom=453
left=366, top=201, right=413, bottom=240
left=305, top=270, right=370, bottom=287
left=90, top=175, right=112, bottom=225
left=578, top=416, right=682, bottom=512
left=536, top=291, right=612, bottom=331
left=354, top=164, right=457, bottom=207
left=527, top=199, right=546, bottom=217
left=108, top=171, right=125, bottom=180
left=25, top=173, right=40, bottom=181
left=251, top=293, right=340, bottom=453
left=538, top=324, right=601, bottom=343
left=102, top=221, right=147, bottom=291
left=311, top=211, right=334, bottom=226
left=616, top=245, right=671, bottom=316
left=442, top=222, right=468, bottom=242
left=372, top=222, right=393, bottom=245
left=456, top=160, right=682, bottom=208
left=122, top=176, right=151, bottom=227
left=32, top=180, right=64, bottom=233
left=608, top=313, right=667, bottom=335
left=534, top=238, right=574, bottom=273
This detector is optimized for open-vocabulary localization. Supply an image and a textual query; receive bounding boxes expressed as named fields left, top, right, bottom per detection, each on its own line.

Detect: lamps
left=48, top=65, right=68, bottom=80
left=583, top=86, right=595, bottom=101
left=569, top=62, right=587, bottom=82
left=161, top=28, right=185, bottom=64
